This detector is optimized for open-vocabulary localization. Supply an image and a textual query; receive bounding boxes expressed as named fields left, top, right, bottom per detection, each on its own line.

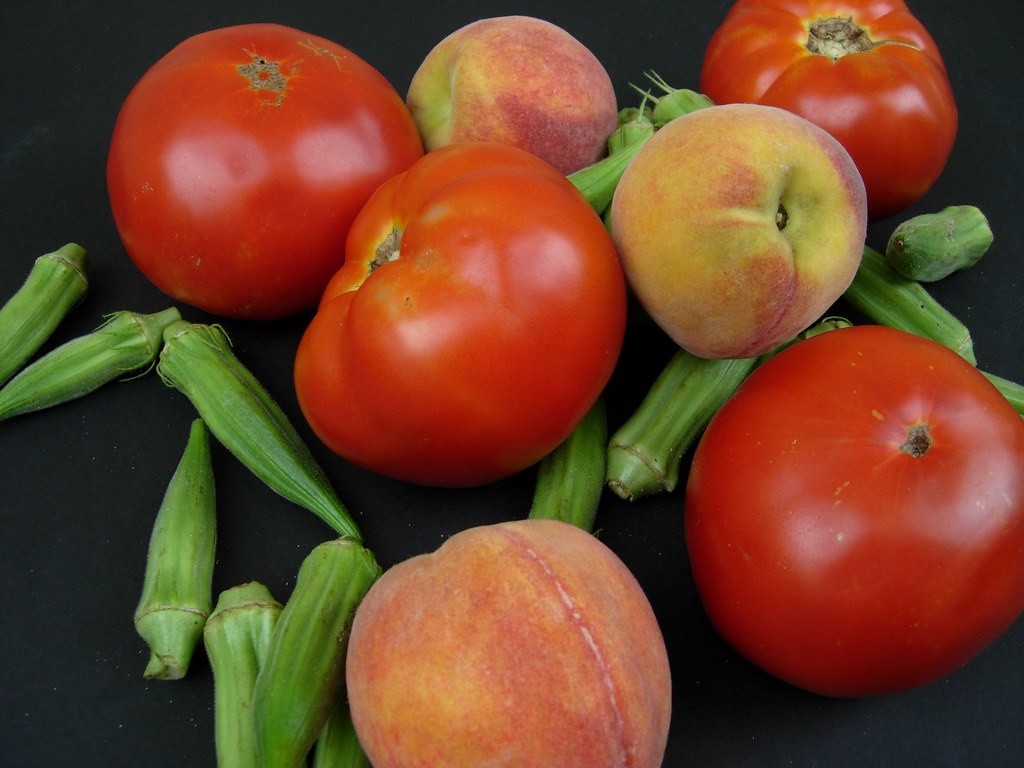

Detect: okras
left=0, top=74, right=1024, bottom=768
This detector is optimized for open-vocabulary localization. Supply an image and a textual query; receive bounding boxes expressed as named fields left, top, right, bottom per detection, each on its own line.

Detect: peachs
left=345, top=518, right=672, bottom=768
left=405, top=16, right=618, bottom=177
left=610, top=102, right=867, bottom=360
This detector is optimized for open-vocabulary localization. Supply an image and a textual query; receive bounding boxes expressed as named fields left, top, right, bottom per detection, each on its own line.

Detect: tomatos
left=293, top=141, right=626, bottom=484
left=699, top=0, right=962, bottom=220
left=107, top=22, right=425, bottom=317
left=685, top=325, right=1024, bottom=698
